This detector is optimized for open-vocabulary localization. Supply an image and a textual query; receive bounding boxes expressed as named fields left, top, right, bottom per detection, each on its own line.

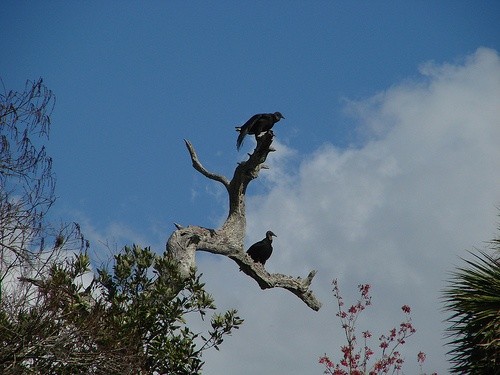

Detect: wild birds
left=235, top=112, right=285, bottom=153
left=238, top=230, right=277, bottom=272
